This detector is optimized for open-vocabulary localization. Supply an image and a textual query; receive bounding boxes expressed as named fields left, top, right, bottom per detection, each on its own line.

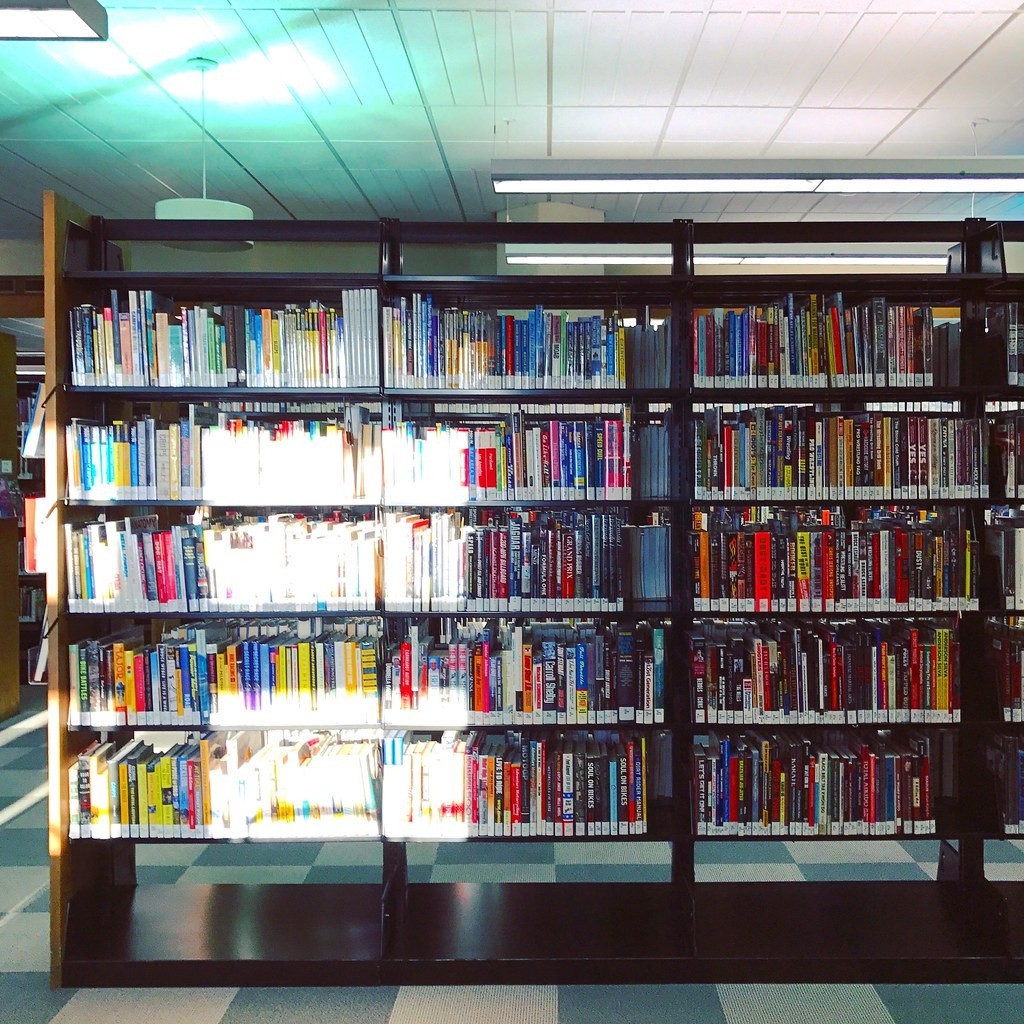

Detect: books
left=15, top=287, right=1024, bottom=838
left=67, top=761, right=81, bottom=838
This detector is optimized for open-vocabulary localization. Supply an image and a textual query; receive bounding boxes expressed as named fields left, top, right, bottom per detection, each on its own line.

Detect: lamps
left=0, top=0, right=107, bottom=41
left=503, top=118, right=978, bottom=265
left=490, top=0, right=1024, bottom=193
left=156, top=59, right=254, bottom=253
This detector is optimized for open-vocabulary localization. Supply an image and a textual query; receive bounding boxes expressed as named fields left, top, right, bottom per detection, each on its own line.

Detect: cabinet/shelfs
left=41, top=188, right=1024, bottom=990
